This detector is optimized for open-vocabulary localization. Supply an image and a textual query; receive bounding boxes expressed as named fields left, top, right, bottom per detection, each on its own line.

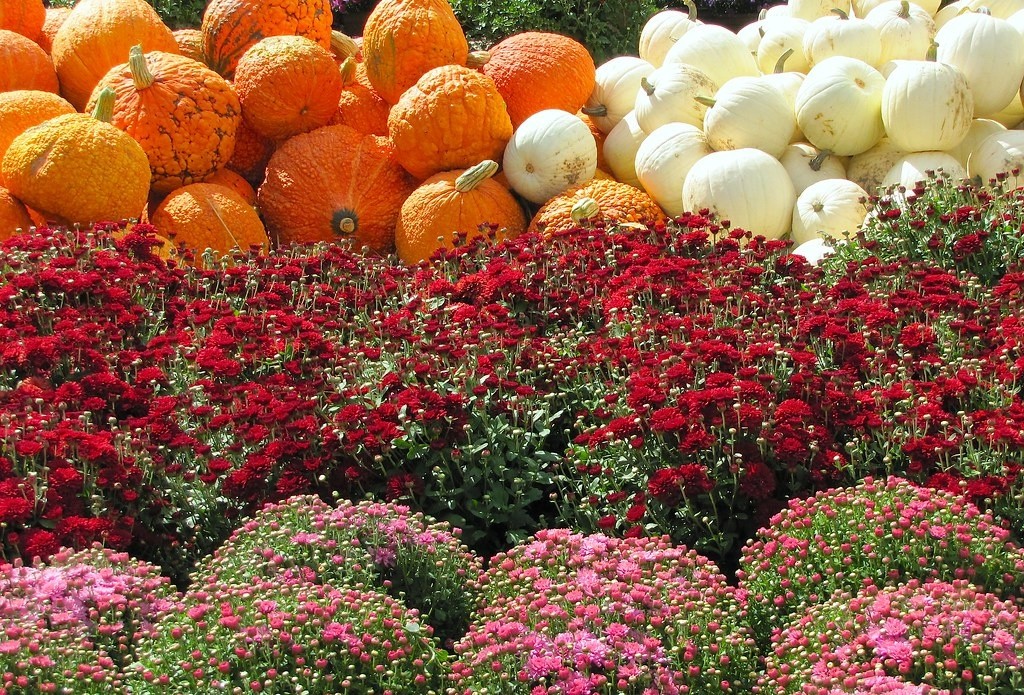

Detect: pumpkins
left=501, top=0, right=1024, bottom=263
left=0, top=0, right=669, bottom=264
